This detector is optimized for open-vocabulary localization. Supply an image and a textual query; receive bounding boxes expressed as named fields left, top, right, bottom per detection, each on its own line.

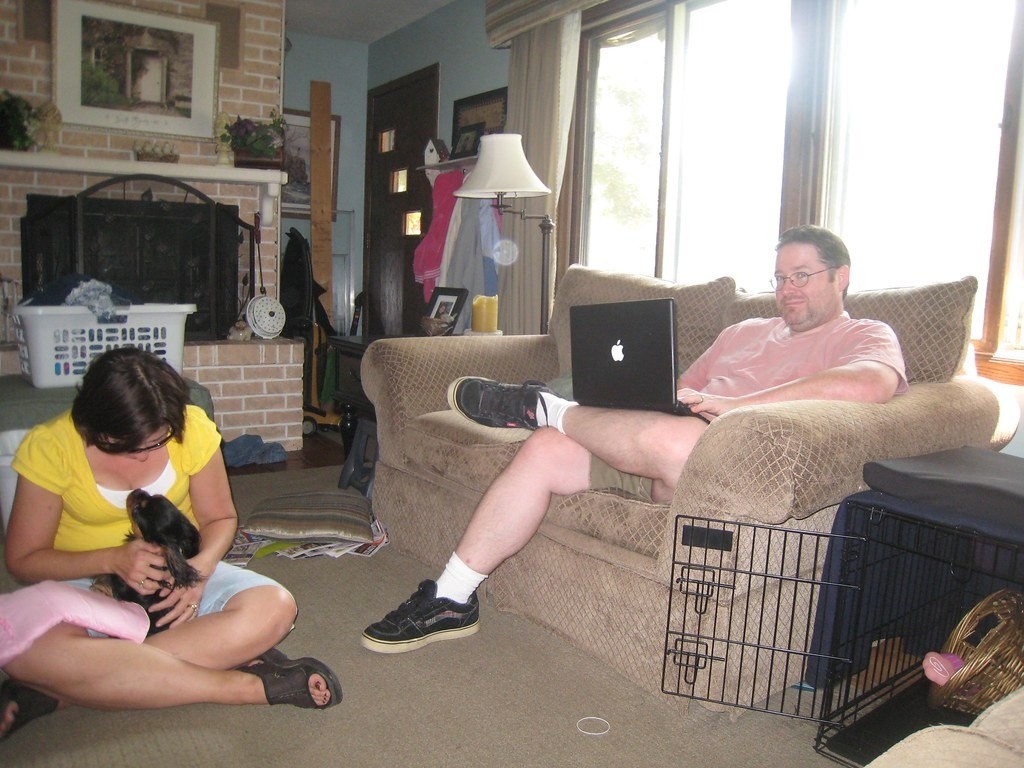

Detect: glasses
left=770, top=264, right=844, bottom=292
left=104, top=423, right=176, bottom=454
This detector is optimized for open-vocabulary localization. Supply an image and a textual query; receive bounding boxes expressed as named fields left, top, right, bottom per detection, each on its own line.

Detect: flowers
left=216, top=104, right=290, bottom=154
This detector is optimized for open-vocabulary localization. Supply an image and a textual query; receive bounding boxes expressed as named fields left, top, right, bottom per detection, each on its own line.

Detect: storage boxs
left=11, top=304, right=198, bottom=390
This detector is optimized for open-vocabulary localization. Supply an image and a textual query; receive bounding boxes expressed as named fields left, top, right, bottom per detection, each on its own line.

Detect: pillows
left=721, top=276, right=980, bottom=384
left=550, top=263, right=735, bottom=379
left=241, top=484, right=376, bottom=544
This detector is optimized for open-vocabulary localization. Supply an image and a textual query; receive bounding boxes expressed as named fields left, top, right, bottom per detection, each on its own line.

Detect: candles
left=465, top=295, right=503, bottom=338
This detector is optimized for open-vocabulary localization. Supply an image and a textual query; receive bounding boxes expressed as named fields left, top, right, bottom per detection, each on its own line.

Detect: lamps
left=452, top=134, right=555, bottom=332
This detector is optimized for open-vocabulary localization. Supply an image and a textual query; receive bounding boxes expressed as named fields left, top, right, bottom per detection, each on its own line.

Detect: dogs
left=92, top=489, right=201, bottom=640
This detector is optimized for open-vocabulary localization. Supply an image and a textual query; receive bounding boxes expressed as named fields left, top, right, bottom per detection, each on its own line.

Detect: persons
left=0, top=346, right=343, bottom=739
left=434, top=303, right=449, bottom=319
left=361, top=225, right=909, bottom=653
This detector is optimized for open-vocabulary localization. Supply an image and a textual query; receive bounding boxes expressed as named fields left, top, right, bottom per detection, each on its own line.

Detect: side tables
left=323, top=333, right=474, bottom=500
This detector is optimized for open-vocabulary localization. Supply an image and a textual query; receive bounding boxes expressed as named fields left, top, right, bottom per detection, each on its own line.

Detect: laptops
left=570, top=297, right=712, bottom=428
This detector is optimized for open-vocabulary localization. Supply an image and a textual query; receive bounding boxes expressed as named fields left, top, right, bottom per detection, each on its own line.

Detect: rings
left=140, top=579, right=145, bottom=587
left=187, top=604, right=199, bottom=612
left=700, top=393, right=704, bottom=403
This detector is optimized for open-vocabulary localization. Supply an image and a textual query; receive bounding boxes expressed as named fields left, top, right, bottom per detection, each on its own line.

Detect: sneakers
left=361, top=580, right=482, bottom=652
left=446, top=376, right=571, bottom=431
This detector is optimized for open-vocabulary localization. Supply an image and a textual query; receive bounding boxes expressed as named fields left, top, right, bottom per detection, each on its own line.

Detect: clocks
left=449, top=121, right=486, bottom=155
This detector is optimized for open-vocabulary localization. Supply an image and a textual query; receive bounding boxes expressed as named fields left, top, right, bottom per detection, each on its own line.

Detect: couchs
left=361, top=263, right=1021, bottom=722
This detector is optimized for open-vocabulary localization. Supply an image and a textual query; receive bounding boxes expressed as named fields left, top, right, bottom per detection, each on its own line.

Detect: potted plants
left=0, top=87, right=63, bottom=152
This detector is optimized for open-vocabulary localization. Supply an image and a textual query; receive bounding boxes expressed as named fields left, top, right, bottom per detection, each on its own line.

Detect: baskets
left=11, top=296, right=199, bottom=388
left=927, top=589, right=1024, bottom=717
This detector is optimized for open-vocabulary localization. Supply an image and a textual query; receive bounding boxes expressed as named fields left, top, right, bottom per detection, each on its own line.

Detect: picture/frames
left=282, top=106, right=342, bottom=221
left=52, top=0, right=221, bottom=143
left=419, top=285, right=468, bottom=337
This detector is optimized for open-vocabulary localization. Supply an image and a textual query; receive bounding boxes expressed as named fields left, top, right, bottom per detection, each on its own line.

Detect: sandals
left=0, top=675, right=59, bottom=741
left=236, top=649, right=344, bottom=710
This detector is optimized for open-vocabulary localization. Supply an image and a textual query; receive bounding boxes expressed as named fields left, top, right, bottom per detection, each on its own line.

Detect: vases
left=232, top=146, right=284, bottom=169
